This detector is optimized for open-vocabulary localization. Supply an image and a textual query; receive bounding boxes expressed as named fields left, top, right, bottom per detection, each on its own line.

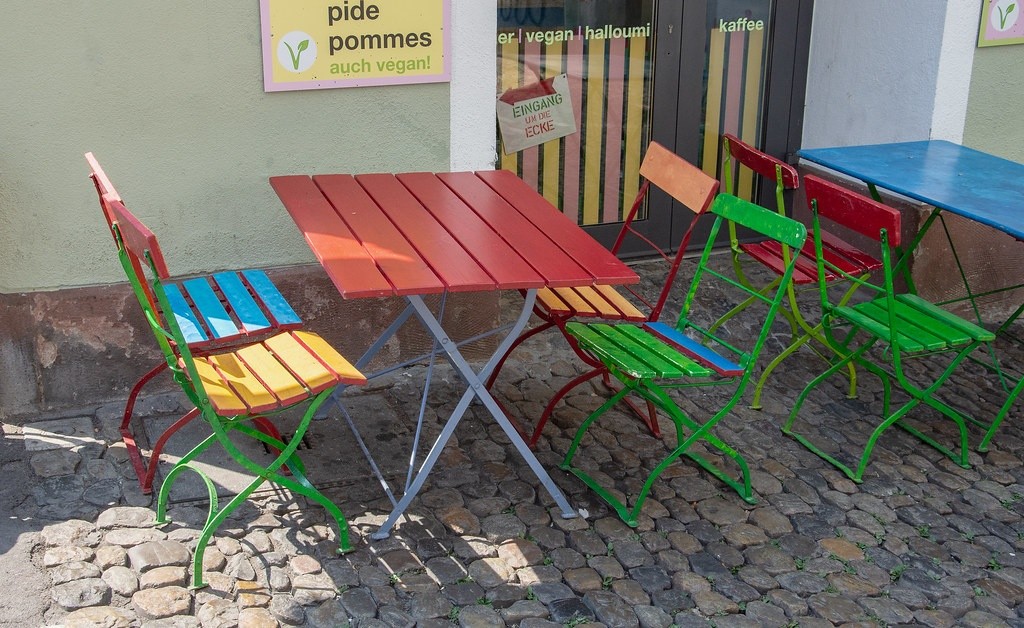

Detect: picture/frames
left=259, top=0, right=452, bottom=92
left=977, top=0, right=1024, bottom=48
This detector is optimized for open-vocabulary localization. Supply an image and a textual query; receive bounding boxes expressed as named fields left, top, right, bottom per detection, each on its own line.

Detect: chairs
left=699, top=131, right=883, bottom=411
left=483, top=139, right=722, bottom=452
left=552, top=191, right=809, bottom=528
left=84, top=151, right=305, bottom=497
left=779, top=172, right=997, bottom=484
left=102, top=193, right=368, bottom=590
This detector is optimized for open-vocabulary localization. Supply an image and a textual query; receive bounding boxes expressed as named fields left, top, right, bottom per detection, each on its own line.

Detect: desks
left=794, top=139, right=1024, bottom=451
left=269, top=169, right=641, bottom=539
